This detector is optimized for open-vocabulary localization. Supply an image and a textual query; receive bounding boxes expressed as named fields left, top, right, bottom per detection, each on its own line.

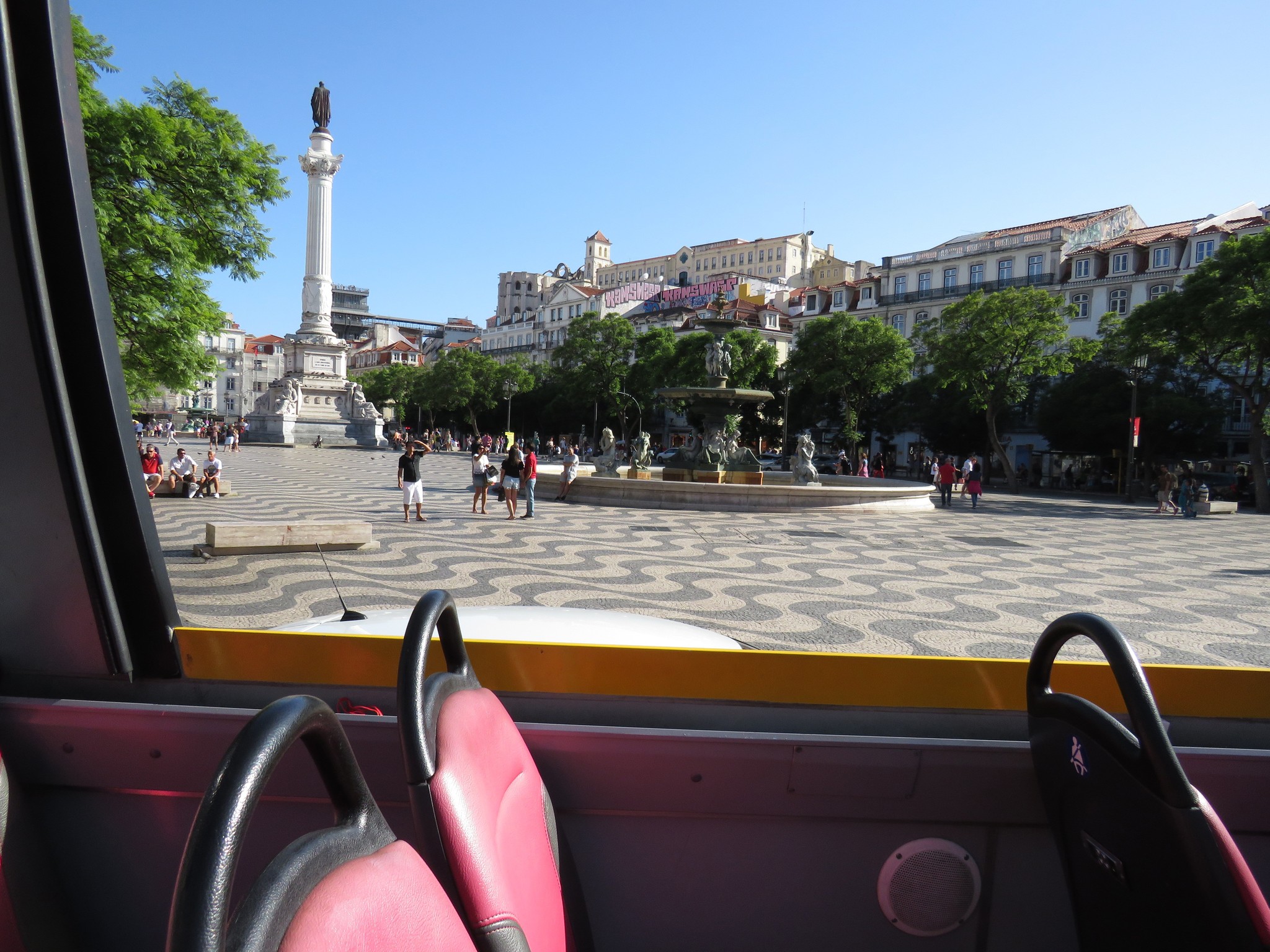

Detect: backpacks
left=846, top=462, right=852, bottom=472
left=1171, top=473, right=1178, bottom=490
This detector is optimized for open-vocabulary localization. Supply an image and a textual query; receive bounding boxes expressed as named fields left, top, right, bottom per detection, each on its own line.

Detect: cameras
left=486, top=448, right=492, bottom=451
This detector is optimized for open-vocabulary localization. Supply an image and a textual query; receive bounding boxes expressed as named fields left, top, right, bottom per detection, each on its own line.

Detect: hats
left=838, top=452, right=845, bottom=456
left=839, top=450, right=845, bottom=452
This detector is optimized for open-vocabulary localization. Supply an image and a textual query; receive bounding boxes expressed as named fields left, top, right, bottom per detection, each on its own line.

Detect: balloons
left=205, top=420, right=210, bottom=425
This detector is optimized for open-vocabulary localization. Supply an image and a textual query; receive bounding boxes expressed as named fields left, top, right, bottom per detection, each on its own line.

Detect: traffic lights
left=689, top=433, right=693, bottom=437
left=535, top=432, right=538, bottom=438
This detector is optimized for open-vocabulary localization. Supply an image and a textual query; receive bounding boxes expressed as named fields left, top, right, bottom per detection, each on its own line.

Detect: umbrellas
left=616, top=440, right=625, bottom=445
left=132, top=419, right=139, bottom=424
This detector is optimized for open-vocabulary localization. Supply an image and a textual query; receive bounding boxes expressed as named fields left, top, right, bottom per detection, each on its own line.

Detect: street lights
left=777, top=365, right=797, bottom=471
left=206, top=382, right=212, bottom=420
left=1125, top=380, right=1137, bottom=504
left=415, top=403, right=421, bottom=439
left=503, top=379, right=519, bottom=432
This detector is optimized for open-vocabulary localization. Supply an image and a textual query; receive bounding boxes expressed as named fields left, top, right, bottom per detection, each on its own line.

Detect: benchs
left=153, top=479, right=238, bottom=496
left=1193, top=501, right=1238, bottom=515
left=588, top=457, right=598, bottom=461
left=289, top=536, right=291, bottom=537
left=193, top=520, right=380, bottom=557
left=951, top=484, right=967, bottom=493
left=558, top=454, right=564, bottom=460
left=451, top=447, right=459, bottom=451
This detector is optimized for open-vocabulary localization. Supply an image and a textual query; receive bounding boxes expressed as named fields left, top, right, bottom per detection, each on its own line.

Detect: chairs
left=394, top=588, right=594, bottom=952
left=1025, top=612, right=1270, bottom=952
left=165, top=694, right=480, bottom=952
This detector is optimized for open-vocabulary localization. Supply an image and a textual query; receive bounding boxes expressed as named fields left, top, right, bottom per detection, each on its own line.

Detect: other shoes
left=198, top=493, right=203, bottom=498
left=416, top=517, right=427, bottom=521
left=149, top=492, right=156, bottom=499
left=520, top=515, right=535, bottom=520
left=473, top=506, right=477, bottom=513
left=506, top=515, right=516, bottom=520
left=481, top=511, right=489, bottom=515
left=404, top=518, right=410, bottom=522
left=1153, top=510, right=1162, bottom=514
left=555, top=495, right=566, bottom=501
left=960, top=494, right=967, bottom=499
left=972, top=507, right=977, bottom=509
left=941, top=504, right=946, bottom=506
left=214, top=493, right=220, bottom=499
left=947, top=502, right=951, bottom=506
left=1174, top=508, right=1179, bottom=515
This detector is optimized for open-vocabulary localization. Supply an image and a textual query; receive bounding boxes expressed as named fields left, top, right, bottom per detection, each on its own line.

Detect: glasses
left=146, top=450, right=154, bottom=453
left=478, top=448, right=485, bottom=450
left=180, top=452, right=185, bottom=454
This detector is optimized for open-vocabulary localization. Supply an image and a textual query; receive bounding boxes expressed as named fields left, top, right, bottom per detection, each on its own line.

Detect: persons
left=393, top=436, right=580, bottom=523
left=669, top=427, right=763, bottom=466
left=762, top=448, right=782, bottom=455
left=616, top=442, right=667, bottom=466
left=353, top=384, right=383, bottom=419
left=133, top=419, right=180, bottom=446
left=274, top=379, right=297, bottom=414
left=593, top=427, right=615, bottom=471
left=137, top=440, right=222, bottom=499
left=795, top=434, right=819, bottom=483
left=314, top=435, right=322, bottom=448
left=311, top=81, right=331, bottom=127
left=704, top=343, right=732, bottom=376
left=1016, top=463, right=1209, bottom=518
left=834, top=449, right=983, bottom=509
left=192, top=395, right=199, bottom=404
left=392, top=427, right=592, bottom=463
left=182, top=418, right=205, bottom=429
left=635, top=432, right=650, bottom=462
left=196, top=416, right=250, bottom=452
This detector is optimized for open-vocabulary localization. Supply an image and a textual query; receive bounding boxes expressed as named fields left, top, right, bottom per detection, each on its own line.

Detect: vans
left=1151, top=471, right=1249, bottom=503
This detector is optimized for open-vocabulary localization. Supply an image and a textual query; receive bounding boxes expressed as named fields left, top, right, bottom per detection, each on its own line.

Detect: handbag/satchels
left=486, top=465, right=498, bottom=486
left=498, top=486, right=505, bottom=502
left=436, top=437, right=440, bottom=442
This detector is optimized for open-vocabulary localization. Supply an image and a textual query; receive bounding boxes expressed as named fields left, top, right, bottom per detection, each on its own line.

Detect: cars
left=763, top=457, right=818, bottom=472
left=656, top=448, right=679, bottom=464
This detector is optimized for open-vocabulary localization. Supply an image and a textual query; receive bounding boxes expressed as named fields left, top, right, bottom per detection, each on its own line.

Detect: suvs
left=755, top=453, right=782, bottom=470
left=814, top=454, right=839, bottom=474
